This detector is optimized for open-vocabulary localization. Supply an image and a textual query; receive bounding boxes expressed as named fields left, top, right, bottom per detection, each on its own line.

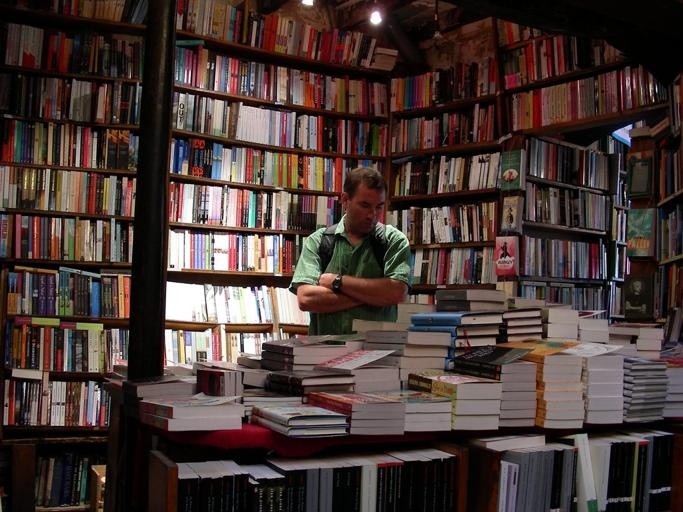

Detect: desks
left=143, top=391, right=472, bottom=452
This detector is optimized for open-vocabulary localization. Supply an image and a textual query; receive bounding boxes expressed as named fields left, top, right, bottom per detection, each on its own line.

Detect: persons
left=288, top=168, right=414, bottom=344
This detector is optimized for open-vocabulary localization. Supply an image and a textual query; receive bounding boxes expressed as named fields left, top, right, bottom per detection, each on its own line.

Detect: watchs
left=331, top=274, right=343, bottom=294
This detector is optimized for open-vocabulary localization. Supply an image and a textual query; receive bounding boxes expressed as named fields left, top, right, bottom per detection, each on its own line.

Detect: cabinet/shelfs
left=0, top=0, right=153, bottom=433
left=177, top=433, right=635, bottom=511
left=164, top=1, right=683, bottom=371
left=3, top=441, right=112, bottom=509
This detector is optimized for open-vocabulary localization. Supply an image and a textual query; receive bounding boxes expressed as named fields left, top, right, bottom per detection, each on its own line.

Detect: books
left=624, top=273, right=653, bottom=320
left=121, top=333, right=404, bottom=436
left=654, top=264, right=683, bottom=319
left=659, top=147, right=683, bottom=200
left=529, top=136, right=610, bottom=191
left=4, top=316, right=129, bottom=373
left=525, top=180, right=606, bottom=231
left=0, top=120, right=139, bottom=172
left=497, top=17, right=551, bottom=45
left=12, top=1, right=147, bottom=25
left=386, top=201, right=497, bottom=242
left=405, top=293, right=436, bottom=304
left=505, top=36, right=630, bottom=89
left=0, top=214, right=133, bottom=263
left=6, top=265, right=132, bottom=319
left=172, top=90, right=387, bottom=158
left=169, top=181, right=345, bottom=230
left=4, top=23, right=144, bottom=81
left=392, top=154, right=501, bottom=197
left=165, top=280, right=312, bottom=325
left=175, top=449, right=458, bottom=512
left=673, top=84, right=680, bottom=133
left=167, top=229, right=306, bottom=276
left=390, top=57, right=495, bottom=112
left=616, top=246, right=626, bottom=279
left=512, top=64, right=669, bottom=137
left=175, top=1, right=398, bottom=71
left=169, top=137, right=345, bottom=194
left=164, top=324, right=289, bottom=366
left=495, top=237, right=520, bottom=277
left=2, top=74, right=142, bottom=126
left=391, top=104, right=495, bottom=153
left=615, top=177, right=628, bottom=206
left=626, top=150, right=653, bottom=198
left=500, top=149, right=527, bottom=191
left=470, top=432, right=675, bottom=511
left=346, top=157, right=385, bottom=174
left=608, top=281, right=621, bottom=314
left=612, top=209, right=626, bottom=243
left=176, top=39, right=388, bottom=118
left=414, top=247, right=497, bottom=284
left=33, top=444, right=107, bottom=512
left=657, top=205, right=683, bottom=261
left=2, top=369, right=111, bottom=427
left=610, top=139, right=628, bottom=170
left=0, top=165, right=136, bottom=217
left=500, top=195, right=524, bottom=236
left=520, top=235, right=608, bottom=281
left=626, top=207, right=655, bottom=257
